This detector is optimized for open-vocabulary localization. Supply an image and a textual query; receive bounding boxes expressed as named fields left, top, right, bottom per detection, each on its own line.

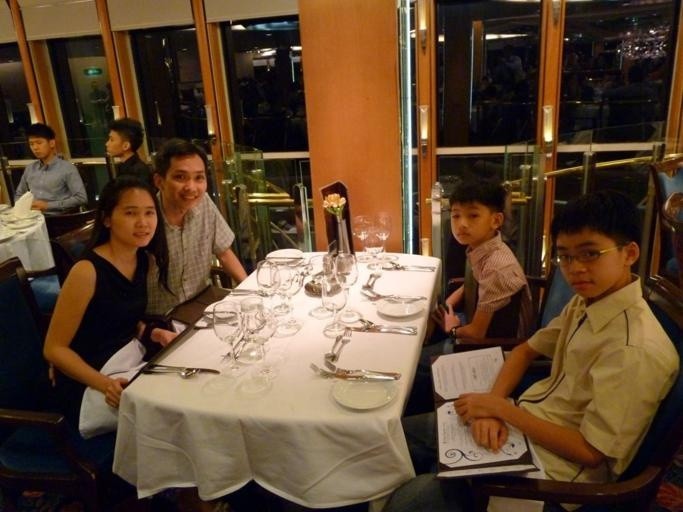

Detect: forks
left=331, top=330, right=352, bottom=363
left=359, top=318, right=417, bottom=336
left=310, top=360, right=401, bottom=383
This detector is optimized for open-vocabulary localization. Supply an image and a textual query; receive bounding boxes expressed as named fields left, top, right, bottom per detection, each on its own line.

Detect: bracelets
left=140, top=324, right=158, bottom=342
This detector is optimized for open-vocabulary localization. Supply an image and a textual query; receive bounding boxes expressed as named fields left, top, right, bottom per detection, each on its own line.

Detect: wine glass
left=211, top=259, right=305, bottom=377
left=351, top=212, right=392, bottom=272
left=333, top=253, right=362, bottom=324
left=321, top=279, right=349, bottom=340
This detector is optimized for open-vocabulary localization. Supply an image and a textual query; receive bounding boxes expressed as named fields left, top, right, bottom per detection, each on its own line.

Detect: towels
left=78, top=338, right=148, bottom=440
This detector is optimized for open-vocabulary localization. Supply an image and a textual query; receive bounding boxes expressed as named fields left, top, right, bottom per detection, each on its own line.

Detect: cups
left=308, top=255, right=332, bottom=284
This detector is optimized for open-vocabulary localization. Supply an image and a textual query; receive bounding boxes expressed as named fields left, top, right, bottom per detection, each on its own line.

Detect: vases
left=338, top=221, right=350, bottom=253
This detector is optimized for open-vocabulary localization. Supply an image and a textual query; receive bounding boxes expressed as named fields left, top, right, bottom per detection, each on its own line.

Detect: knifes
left=148, top=363, right=221, bottom=374
left=344, top=327, right=416, bottom=336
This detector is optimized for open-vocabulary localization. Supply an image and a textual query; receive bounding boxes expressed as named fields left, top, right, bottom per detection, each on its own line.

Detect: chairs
left=650, top=154, right=683, bottom=288
left=29, top=209, right=100, bottom=317
left=8, top=161, right=88, bottom=207
left=0, top=257, right=115, bottom=512
left=663, top=192, right=683, bottom=288
left=50, top=222, right=233, bottom=334
left=470, top=276, right=683, bottom=512
left=446, top=261, right=577, bottom=353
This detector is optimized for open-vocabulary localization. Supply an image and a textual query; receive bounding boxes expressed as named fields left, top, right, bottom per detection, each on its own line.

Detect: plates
left=264, top=248, right=305, bottom=266
left=329, top=376, right=401, bottom=410
left=375, top=295, right=425, bottom=317
left=0, top=201, right=41, bottom=241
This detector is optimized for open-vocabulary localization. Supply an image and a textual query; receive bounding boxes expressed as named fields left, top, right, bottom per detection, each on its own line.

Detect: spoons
left=325, top=328, right=342, bottom=363
left=362, top=273, right=381, bottom=290
left=141, top=368, right=201, bottom=379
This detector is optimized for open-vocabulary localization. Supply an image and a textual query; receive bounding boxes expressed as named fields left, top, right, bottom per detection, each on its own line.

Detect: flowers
left=323, top=193, right=346, bottom=221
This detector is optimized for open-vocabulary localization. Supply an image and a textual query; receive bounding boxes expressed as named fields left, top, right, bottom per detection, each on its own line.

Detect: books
left=427, top=344, right=542, bottom=481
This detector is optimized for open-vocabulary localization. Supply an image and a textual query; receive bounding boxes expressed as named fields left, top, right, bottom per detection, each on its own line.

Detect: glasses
left=550, top=243, right=626, bottom=265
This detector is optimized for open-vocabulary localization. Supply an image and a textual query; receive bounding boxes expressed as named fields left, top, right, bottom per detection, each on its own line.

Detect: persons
left=10, top=123, right=89, bottom=239
left=40, top=172, right=179, bottom=464
left=103, top=116, right=153, bottom=194
left=369, top=185, right=682, bottom=512
left=407, top=175, right=540, bottom=389
left=142, top=137, right=250, bottom=330
